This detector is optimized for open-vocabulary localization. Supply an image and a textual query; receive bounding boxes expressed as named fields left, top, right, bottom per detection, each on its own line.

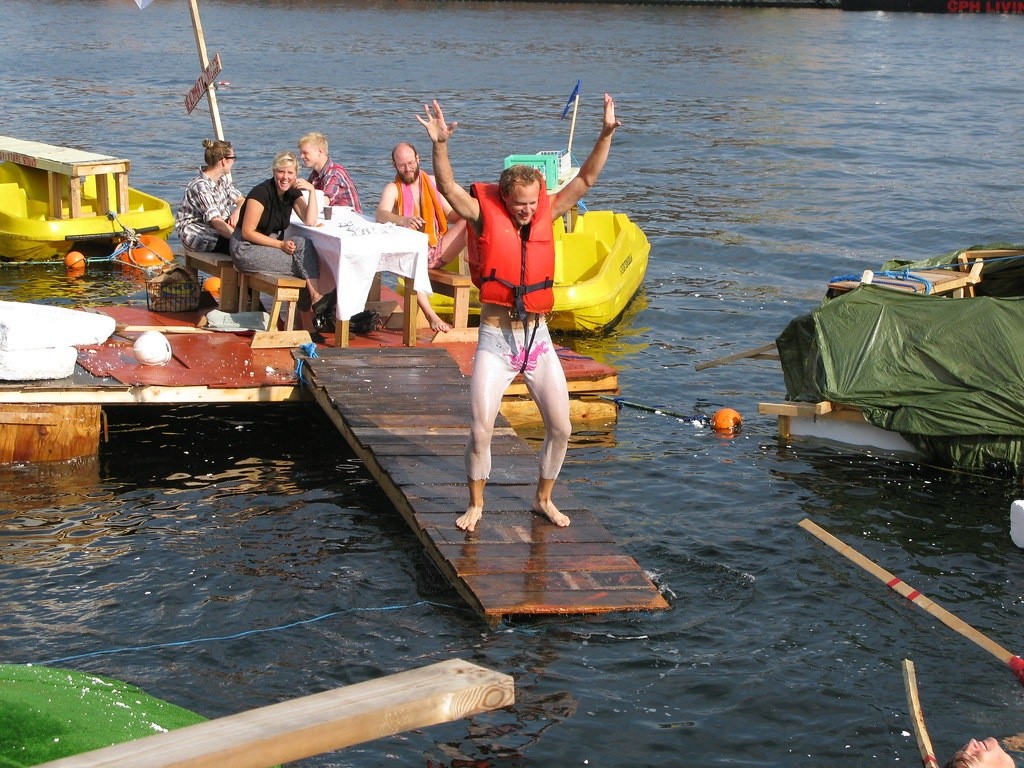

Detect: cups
left=323, top=206, right=332, bottom=220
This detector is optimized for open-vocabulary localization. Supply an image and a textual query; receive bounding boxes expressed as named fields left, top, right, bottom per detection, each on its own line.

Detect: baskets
left=144, top=276, right=203, bottom=312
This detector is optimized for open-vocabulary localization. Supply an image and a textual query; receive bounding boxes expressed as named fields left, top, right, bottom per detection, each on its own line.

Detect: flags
left=561, top=79, right=581, bottom=121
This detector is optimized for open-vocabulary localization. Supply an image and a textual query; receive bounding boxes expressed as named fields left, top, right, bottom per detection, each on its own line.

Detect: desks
left=288, top=207, right=432, bottom=347
left=545, top=167, right=581, bottom=233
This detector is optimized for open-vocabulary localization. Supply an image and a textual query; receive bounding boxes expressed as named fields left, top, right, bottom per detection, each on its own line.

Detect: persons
left=297, top=131, right=364, bottom=214
left=175, top=139, right=269, bottom=313
left=230, top=151, right=328, bottom=344
left=375, top=141, right=468, bottom=333
left=415, top=93, right=621, bottom=531
left=942, top=732, right=1024, bottom=768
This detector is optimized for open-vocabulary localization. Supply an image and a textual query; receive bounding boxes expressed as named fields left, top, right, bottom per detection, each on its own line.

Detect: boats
left=396, top=152, right=652, bottom=339
left=0, top=133, right=176, bottom=269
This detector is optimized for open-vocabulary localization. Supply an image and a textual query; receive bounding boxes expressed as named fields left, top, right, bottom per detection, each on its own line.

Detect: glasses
left=219, top=156, right=236, bottom=161
left=397, top=160, right=416, bottom=167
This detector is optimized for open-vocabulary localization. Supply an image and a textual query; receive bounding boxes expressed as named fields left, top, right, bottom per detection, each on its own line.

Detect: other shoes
left=310, top=331, right=325, bottom=344
left=312, top=289, right=336, bottom=314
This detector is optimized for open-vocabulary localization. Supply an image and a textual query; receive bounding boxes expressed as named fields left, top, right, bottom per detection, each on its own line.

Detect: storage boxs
left=505, top=153, right=559, bottom=189
left=535, top=150, right=573, bottom=169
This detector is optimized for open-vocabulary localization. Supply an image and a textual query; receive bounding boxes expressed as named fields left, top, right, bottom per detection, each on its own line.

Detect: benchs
left=430, top=269, right=472, bottom=327
left=178, top=244, right=238, bottom=311
left=828, top=267, right=981, bottom=307
left=237, top=269, right=313, bottom=347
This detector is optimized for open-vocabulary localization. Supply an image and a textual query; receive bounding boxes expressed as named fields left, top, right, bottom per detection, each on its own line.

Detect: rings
left=298, top=181, right=300, bottom=183
